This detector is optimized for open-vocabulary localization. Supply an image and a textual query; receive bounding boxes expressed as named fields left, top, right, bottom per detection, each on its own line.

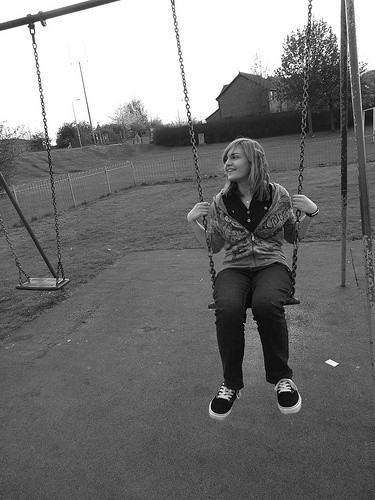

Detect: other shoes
left=273, top=378, right=303, bottom=415
left=208, top=381, right=241, bottom=420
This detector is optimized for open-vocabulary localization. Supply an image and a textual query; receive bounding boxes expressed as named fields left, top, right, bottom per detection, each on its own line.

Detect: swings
left=170, top=0, right=313, bottom=309
left=1, top=23, right=69, bottom=292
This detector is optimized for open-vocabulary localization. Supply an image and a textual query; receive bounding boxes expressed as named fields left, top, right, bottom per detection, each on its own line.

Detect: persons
left=186, top=138, right=318, bottom=420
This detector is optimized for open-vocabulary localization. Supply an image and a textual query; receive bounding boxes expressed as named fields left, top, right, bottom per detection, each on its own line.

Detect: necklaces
left=246, top=200, right=249, bottom=203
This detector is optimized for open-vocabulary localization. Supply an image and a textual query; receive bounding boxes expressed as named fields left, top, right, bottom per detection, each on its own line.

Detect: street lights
left=71, top=98, right=82, bottom=147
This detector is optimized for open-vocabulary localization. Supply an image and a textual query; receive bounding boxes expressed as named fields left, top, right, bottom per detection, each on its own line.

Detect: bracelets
left=305, top=209, right=319, bottom=217
